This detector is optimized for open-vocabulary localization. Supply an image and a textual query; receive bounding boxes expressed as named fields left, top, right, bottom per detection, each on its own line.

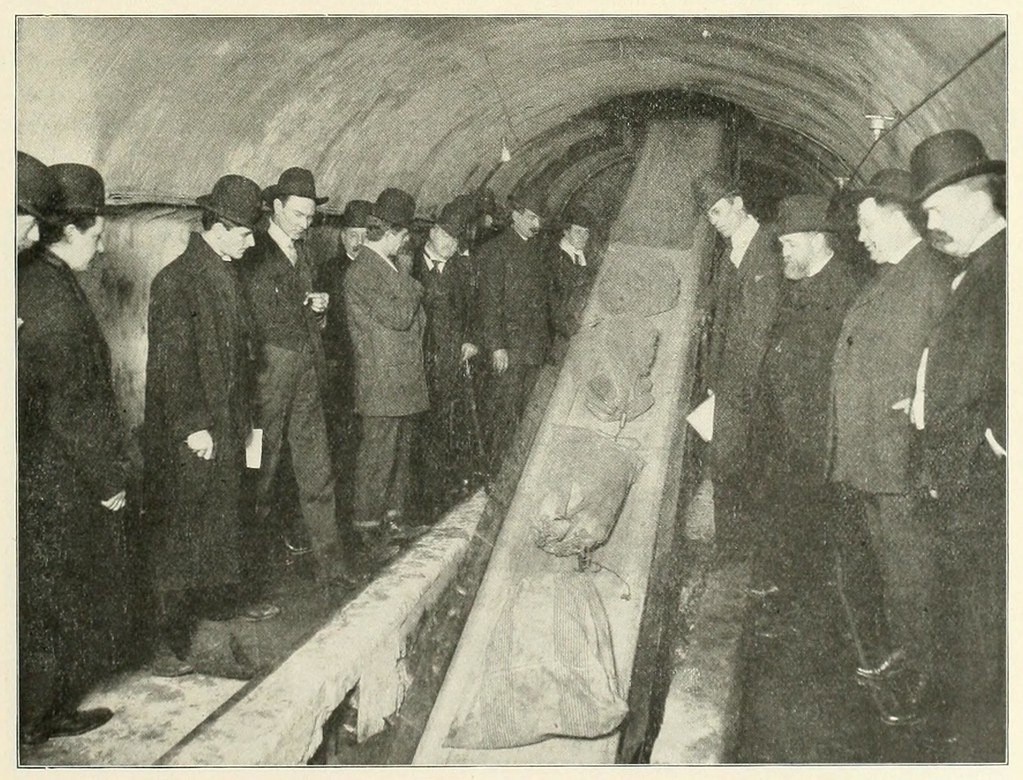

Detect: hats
left=16, top=127, right=1013, bottom=238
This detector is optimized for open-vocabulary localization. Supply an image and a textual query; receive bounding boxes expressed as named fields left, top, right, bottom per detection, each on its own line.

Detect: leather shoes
left=18, top=468, right=1005, bottom=751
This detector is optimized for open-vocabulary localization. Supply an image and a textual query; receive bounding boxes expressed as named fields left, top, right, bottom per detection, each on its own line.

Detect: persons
left=756, top=195, right=880, bottom=652
left=696, top=161, right=795, bottom=603
left=19, top=160, right=199, bottom=677
left=413, top=196, right=478, bottom=517
left=822, top=162, right=951, bottom=731
left=236, top=164, right=379, bottom=596
left=902, top=127, right=1004, bottom=762
left=17, top=142, right=116, bottom=744
left=127, top=170, right=284, bottom=628
left=475, top=186, right=604, bottom=480
left=343, top=186, right=435, bottom=564
left=550, top=205, right=601, bottom=364
left=283, top=198, right=389, bottom=558
